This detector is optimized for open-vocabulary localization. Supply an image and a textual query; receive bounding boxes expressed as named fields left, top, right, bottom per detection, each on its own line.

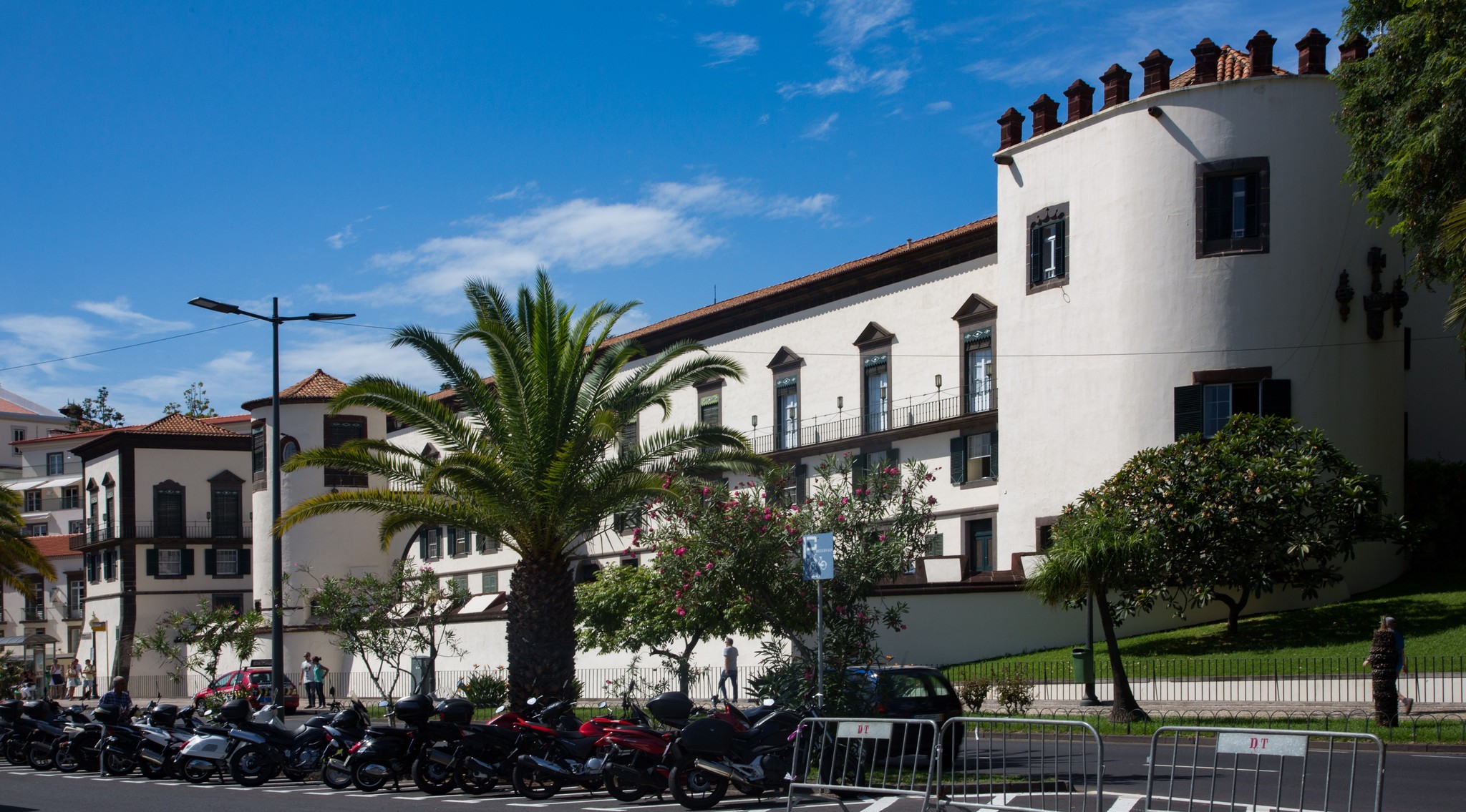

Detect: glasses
left=304, top=656, right=310, bottom=657
left=725, top=641, right=728, bottom=643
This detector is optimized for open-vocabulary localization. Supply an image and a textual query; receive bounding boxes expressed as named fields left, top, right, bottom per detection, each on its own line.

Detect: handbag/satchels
left=48, top=676, right=51, bottom=682
left=75, top=678, right=82, bottom=685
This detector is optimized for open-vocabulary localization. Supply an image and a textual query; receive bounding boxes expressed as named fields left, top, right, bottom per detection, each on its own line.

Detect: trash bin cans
left=1071, top=647, right=1096, bottom=684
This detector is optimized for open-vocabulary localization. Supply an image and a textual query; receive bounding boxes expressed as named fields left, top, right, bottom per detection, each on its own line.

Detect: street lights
left=89, top=612, right=100, bottom=700
left=426, top=587, right=439, bottom=698
left=188, top=296, right=357, bottom=713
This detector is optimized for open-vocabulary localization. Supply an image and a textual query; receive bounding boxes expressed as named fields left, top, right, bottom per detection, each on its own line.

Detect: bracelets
left=1367, top=659, right=1370, bottom=661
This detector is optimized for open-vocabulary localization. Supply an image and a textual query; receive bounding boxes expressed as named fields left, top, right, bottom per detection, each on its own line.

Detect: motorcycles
left=0, top=664, right=871, bottom=811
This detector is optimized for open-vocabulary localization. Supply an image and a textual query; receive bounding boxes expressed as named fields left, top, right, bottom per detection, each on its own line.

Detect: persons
left=97, top=676, right=132, bottom=777
left=312, top=656, right=329, bottom=709
left=67, top=663, right=78, bottom=701
left=79, top=659, right=95, bottom=701
left=65, top=659, right=85, bottom=699
left=719, top=638, right=739, bottom=703
left=20, top=669, right=36, bottom=700
left=299, top=652, right=316, bottom=709
left=3, top=662, right=27, bottom=699
left=1363, top=616, right=1414, bottom=716
left=45, top=666, right=51, bottom=690
left=49, top=658, right=65, bottom=700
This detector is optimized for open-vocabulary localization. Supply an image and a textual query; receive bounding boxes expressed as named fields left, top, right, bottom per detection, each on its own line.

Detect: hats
left=1379, top=617, right=1395, bottom=625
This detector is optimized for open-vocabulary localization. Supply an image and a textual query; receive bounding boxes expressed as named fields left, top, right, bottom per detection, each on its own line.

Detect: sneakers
left=1405, top=698, right=1413, bottom=715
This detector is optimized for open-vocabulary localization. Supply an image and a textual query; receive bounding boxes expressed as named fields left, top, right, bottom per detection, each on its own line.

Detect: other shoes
left=314, top=704, right=326, bottom=709
left=722, top=699, right=728, bottom=701
left=79, top=698, right=85, bottom=700
left=304, top=705, right=315, bottom=709
left=88, top=697, right=91, bottom=700
left=21, top=697, right=31, bottom=700
left=730, top=700, right=738, bottom=703
left=58, top=697, right=63, bottom=700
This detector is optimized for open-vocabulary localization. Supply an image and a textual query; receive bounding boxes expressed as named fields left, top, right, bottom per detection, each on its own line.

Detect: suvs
left=737, top=665, right=963, bottom=770
